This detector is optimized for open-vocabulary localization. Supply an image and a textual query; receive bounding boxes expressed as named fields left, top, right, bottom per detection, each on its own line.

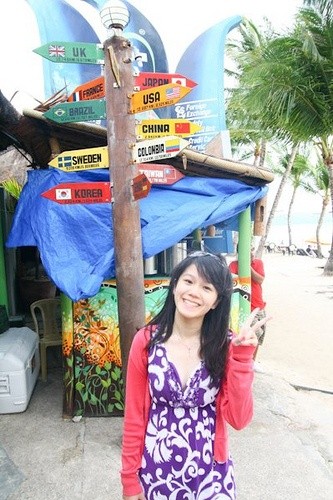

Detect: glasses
left=189, top=250, right=226, bottom=269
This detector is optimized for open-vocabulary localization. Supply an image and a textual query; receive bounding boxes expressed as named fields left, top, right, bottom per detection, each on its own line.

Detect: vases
left=19, top=275, right=56, bottom=314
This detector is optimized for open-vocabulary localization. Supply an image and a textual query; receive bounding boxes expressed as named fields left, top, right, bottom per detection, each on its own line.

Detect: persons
left=229, top=243, right=267, bottom=360
left=121, top=249, right=271, bottom=500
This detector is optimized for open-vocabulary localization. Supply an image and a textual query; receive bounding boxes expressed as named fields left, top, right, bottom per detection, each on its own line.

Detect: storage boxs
left=0, top=327, right=41, bottom=414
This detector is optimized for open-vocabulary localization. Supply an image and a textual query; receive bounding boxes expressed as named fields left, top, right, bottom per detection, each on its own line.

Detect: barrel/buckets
left=142, top=238, right=188, bottom=278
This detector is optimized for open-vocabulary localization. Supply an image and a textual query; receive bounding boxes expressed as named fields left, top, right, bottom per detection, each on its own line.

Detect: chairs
left=30, top=297, right=63, bottom=384
left=263, top=242, right=328, bottom=260
